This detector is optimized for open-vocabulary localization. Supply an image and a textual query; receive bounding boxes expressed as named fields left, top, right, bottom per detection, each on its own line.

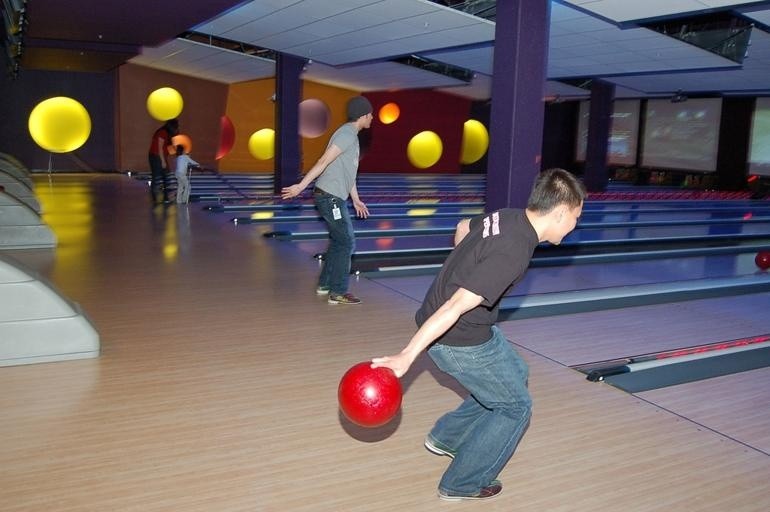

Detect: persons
left=149, top=119, right=180, bottom=204
left=172, top=144, right=200, bottom=204
left=280, top=95, right=374, bottom=304
left=370, top=168, right=585, bottom=501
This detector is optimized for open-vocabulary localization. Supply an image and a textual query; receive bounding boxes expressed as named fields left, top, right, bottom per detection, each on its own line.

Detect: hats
left=346, top=96, right=372, bottom=117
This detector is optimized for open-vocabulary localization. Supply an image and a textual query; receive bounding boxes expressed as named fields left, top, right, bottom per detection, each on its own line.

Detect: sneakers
left=316, top=286, right=330, bottom=293
left=328, top=292, right=361, bottom=304
left=437, top=479, right=504, bottom=500
left=424, top=437, right=458, bottom=458
